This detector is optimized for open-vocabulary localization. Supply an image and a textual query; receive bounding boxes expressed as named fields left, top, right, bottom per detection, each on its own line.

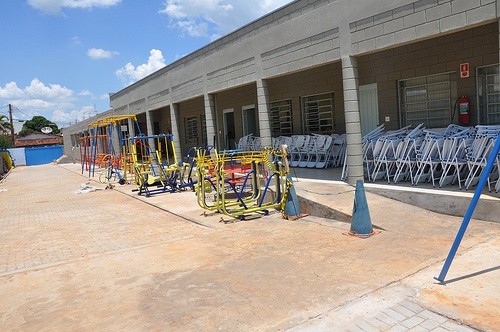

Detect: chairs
left=234, top=120, right=500, bottom=193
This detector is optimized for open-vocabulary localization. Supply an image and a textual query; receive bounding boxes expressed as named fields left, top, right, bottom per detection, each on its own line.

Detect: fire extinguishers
left=450, top=94, right=470, bottom=125
